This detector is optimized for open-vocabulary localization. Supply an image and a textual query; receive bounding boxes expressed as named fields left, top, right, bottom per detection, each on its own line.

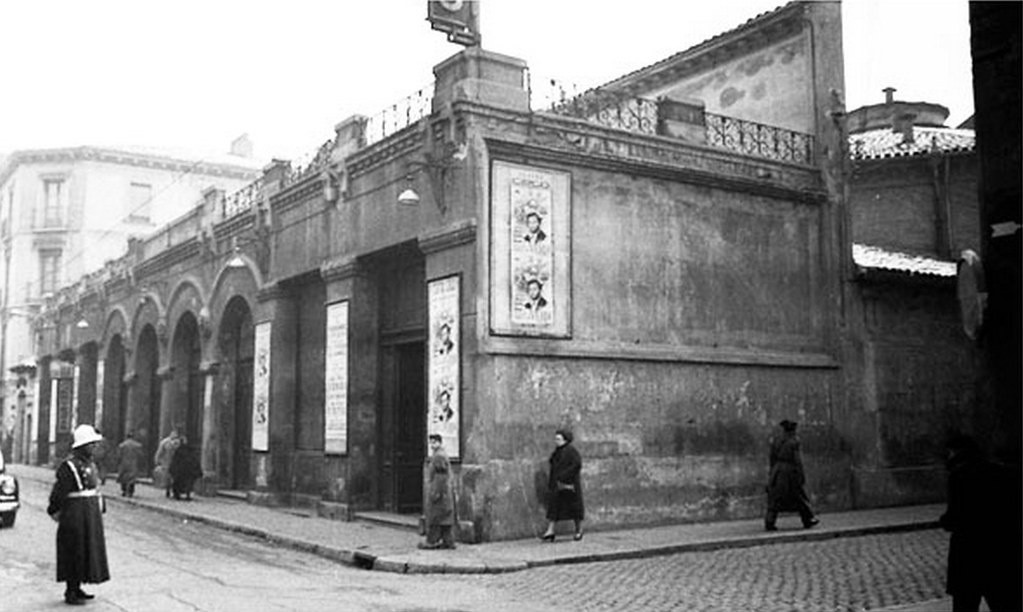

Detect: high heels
left=537, top=533, right=555, bottom=543
left=573, top=529, right=584, bottom=540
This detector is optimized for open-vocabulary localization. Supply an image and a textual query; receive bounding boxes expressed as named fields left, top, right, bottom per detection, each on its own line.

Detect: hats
left=70, top=423, right=103, bottom=449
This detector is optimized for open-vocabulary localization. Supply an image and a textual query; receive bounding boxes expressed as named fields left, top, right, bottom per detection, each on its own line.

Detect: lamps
left=399, top=161, right=463, bottom=206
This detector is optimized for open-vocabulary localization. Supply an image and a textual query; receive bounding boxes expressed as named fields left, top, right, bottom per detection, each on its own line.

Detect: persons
left=939, top=439, right=1022, bottom=612
left=114, top=432, right=145, bottom=498
left=524, top=212, right=546, bottom=243
left=538, top=430, right=585, bottom=542
left=764, top=420, right=818, bottom=531
left=154, top=429, right=204, bottom=501
left=437, top=323, right=452, bottom=354
left=90, top=429, right=111, bottom=485
left=417, top=434, right=457, bottom=549
left=439, top=390, right=452, bottom=421
left=47, top=424, right=110, bottom=606
left=524, top=280, right=546, bottom=310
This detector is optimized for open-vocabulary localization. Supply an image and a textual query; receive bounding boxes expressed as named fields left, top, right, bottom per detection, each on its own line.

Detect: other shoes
left=127, top=494, right=132, bottom=497
left=122, top=493, right=125, bottom=496
left=166, top=490, right=169, bottom=497
left=77, top=589, right=95, bottom=598
left=437, top=543, right=456, bottom=550
left=64, top=595, right=87, bottom=605
left=803, top=519, right=819, bottom=528
left=765, top=526, right=778, bottom=531
left=172, top=494, right=181, bottom=500
left=417, top=543, right=436, bottom=549
left=184, top=497, right=193, bottom=500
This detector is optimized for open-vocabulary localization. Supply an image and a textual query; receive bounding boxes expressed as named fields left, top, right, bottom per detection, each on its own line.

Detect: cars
left=0, top=451, right=21, bottom=528
